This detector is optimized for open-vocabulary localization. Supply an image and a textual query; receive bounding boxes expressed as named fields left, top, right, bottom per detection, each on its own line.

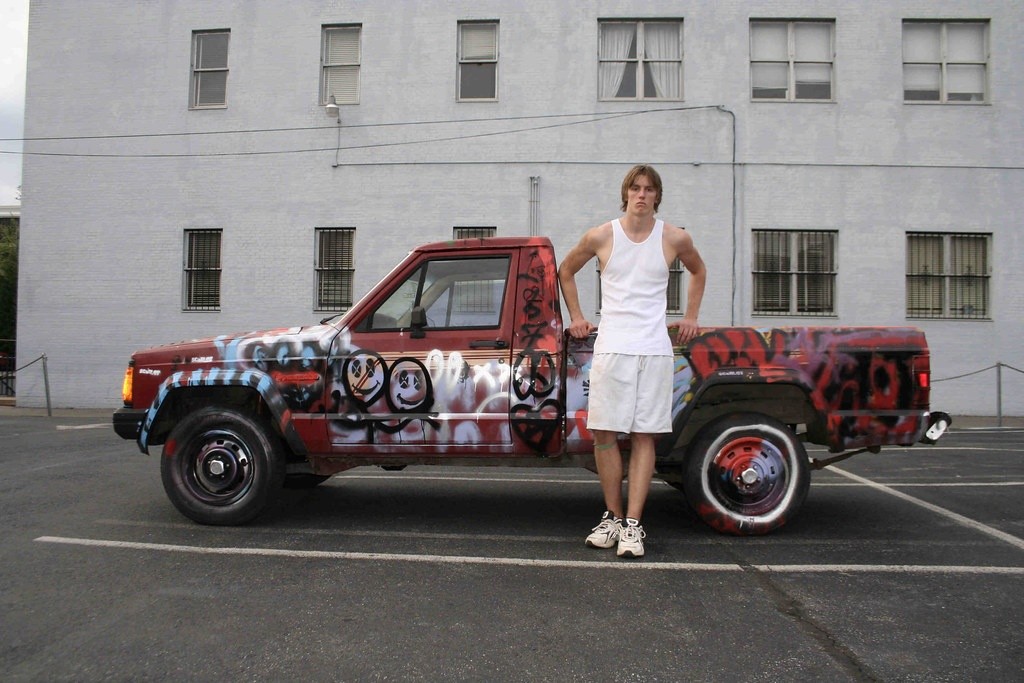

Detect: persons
left=557, top=165, right=707, bottom=557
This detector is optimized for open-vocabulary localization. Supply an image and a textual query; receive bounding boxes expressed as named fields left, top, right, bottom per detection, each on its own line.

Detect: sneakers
left=616, top=516, right=646, bottom=556
left=585, top=509, right=622, bottom=548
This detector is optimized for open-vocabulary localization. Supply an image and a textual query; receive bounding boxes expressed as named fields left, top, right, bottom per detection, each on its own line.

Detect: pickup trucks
left=112, top=238, right=954, bottom=540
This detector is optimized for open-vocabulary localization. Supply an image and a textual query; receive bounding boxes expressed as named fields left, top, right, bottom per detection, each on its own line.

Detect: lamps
left=325, top=93, right=341, bottom=119
